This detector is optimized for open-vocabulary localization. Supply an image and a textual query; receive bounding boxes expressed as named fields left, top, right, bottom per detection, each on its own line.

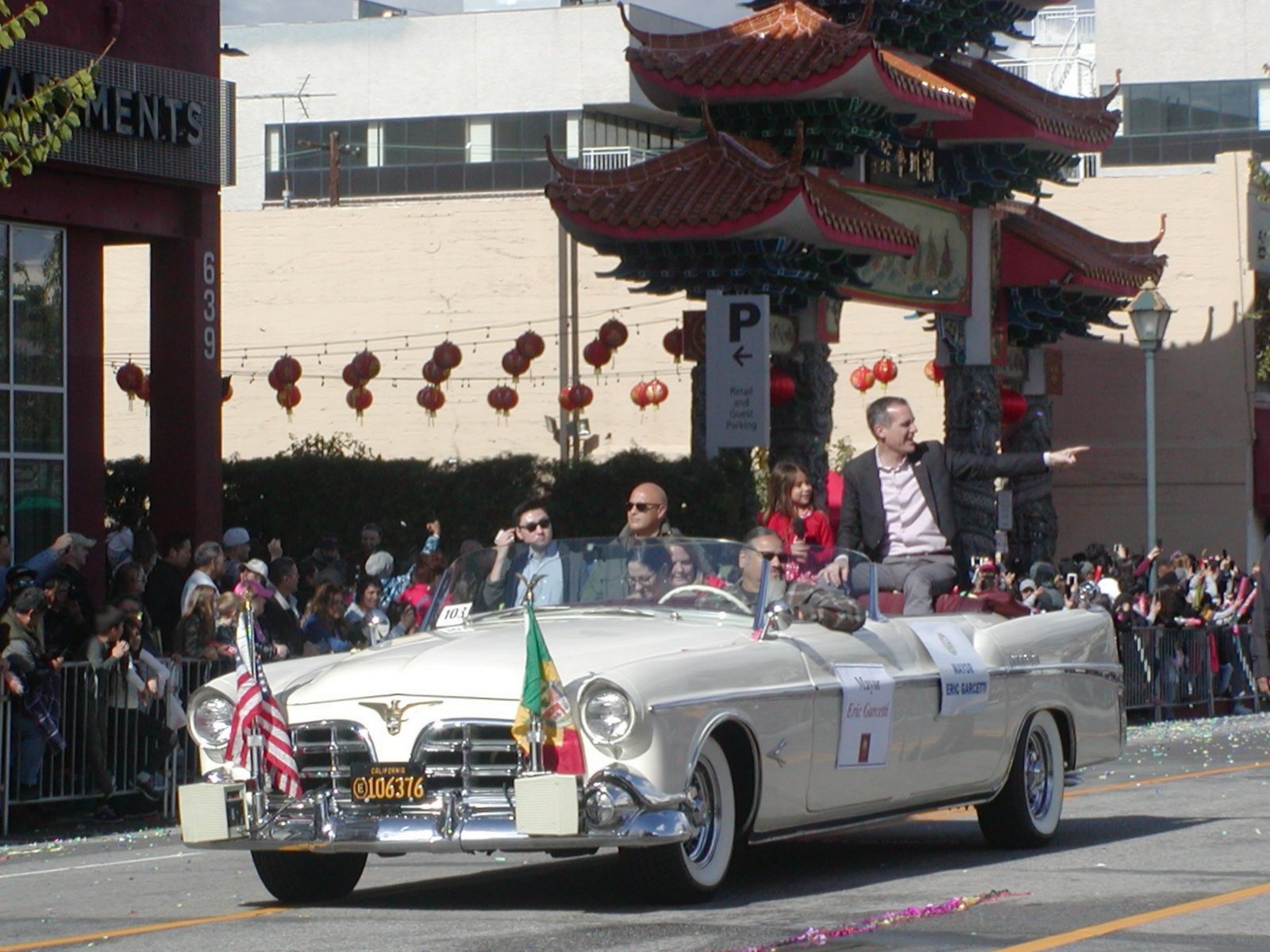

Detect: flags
left=222, top=606, right=303, bottom=801
left=512, top=600, right=586, bottom=775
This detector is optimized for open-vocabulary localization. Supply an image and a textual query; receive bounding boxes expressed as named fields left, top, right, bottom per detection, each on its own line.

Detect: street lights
left=1124, top=274, right=1177, bottom=594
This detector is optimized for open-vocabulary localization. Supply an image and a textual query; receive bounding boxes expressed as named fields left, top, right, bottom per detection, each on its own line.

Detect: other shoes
left=0, top=776, right=163, bottom=843
left=1221, top=687, right=1246, bottom=699
left=1233, top=702, right=1254, bottom=715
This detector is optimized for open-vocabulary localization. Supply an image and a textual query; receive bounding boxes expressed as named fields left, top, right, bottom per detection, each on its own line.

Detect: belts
left=894, top=549, right=954, bottom=557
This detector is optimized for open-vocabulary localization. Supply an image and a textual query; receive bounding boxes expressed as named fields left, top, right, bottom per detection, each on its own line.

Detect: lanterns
left=417, top=339, right=463, bottom=426
left=116, top=363, right=150, bottom=414
left=851, top=356, right=898, bottom=395
left=769, top=369, right=795, bottom=404
left=925, top=358, right=944, bottom=388
left=631, top=329, right=685, bottom=412
left=343, top=350, right=380, bottom=425
left=557, top=319, right=628, bottom=411
left=1000, top=383, right=1029, bottom=430
left=223, top=355, right=302, bottom=414
left=488, top=331, right=545, bottom=421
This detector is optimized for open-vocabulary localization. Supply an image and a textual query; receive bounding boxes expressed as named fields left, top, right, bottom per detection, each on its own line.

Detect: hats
left=365, top=551, right=393, bottom=577
left=55, top=534, right=97, bottom=547
left=1019, top=578, right=1036, bottom=592
left=223, top=528, right=249, bottom=548
left=236, top=559, right=269, bottom=581
left=235, top=580, right=273, bottom=599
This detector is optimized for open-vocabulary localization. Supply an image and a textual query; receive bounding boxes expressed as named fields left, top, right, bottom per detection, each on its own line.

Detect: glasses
left=624, top=567, right=660, bottom=586
left=517, top=517, right=551, bottom=533
left=625, top=502, right=661, bottom=512
left=746, top=548, right=788, bottom=564
left=331, top=599, right=344, bottom=604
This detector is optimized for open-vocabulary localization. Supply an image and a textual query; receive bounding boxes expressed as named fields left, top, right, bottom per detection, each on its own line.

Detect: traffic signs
left=705, top=288, right=771, bottom=452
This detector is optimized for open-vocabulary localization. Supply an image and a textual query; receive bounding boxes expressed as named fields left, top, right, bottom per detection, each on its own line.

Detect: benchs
left=857, top=590, right=993, bottom=615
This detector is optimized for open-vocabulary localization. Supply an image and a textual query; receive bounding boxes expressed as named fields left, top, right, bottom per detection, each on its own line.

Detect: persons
left=0, top=398, right=1259, bottom=839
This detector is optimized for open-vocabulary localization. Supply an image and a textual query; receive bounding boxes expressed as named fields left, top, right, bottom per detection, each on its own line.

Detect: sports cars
left=174, top=535, right=1131, bottom=906
